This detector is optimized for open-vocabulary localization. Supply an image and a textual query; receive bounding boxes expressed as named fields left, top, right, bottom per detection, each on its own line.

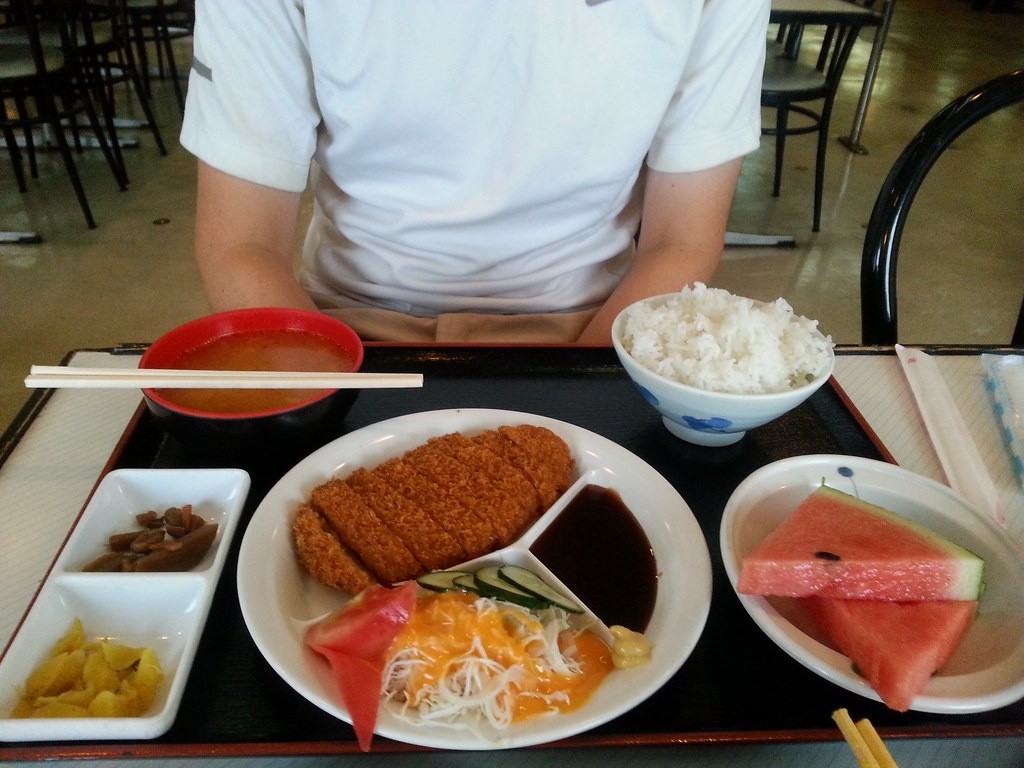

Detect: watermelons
left=736, top=486, right=986, bottom=603
left=805, top=596, right=983, bottom=711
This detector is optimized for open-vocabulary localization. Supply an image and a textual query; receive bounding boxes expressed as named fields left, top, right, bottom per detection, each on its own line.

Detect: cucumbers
left=415, top=565, right=587, bottom=614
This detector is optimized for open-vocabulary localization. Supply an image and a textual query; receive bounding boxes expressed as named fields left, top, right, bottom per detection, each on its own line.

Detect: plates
left=720, top=454, right=1023, bottom=714
left=237, top=408, right=713, bottom=751
left=1, top=468, right=251, bottom=742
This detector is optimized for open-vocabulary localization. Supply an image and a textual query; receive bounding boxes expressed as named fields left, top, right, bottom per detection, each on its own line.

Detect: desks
left=0, top=335, right=1023, bottom=766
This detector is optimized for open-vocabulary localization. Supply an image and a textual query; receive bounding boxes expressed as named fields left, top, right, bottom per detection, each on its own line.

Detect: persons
left=177, top=0, right=773, bottom=343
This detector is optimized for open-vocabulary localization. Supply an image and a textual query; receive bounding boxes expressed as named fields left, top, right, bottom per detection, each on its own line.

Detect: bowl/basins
left=138, top=307, right=364, bottom=444
left=611, top=292, right=835, bottom=447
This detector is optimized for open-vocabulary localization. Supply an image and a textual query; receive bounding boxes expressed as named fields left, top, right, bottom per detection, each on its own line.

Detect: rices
left=622, top=279, right=835, bottom=394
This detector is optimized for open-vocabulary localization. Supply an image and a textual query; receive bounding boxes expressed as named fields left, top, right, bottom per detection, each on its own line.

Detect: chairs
left=861, top=72, right=1024, bottom=345
left=759, top=1, right=897, bottom=235
left=0, top=0, right=193, bottom=230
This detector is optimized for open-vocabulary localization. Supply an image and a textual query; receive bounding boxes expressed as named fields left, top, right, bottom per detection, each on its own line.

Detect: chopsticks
left=831, top=707, right=899, bottom=768
left=25, top=364, right=424, bottom=388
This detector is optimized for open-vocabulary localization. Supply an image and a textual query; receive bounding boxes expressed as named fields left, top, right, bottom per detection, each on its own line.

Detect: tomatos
left=305, top=579, right=416, bottom=751
